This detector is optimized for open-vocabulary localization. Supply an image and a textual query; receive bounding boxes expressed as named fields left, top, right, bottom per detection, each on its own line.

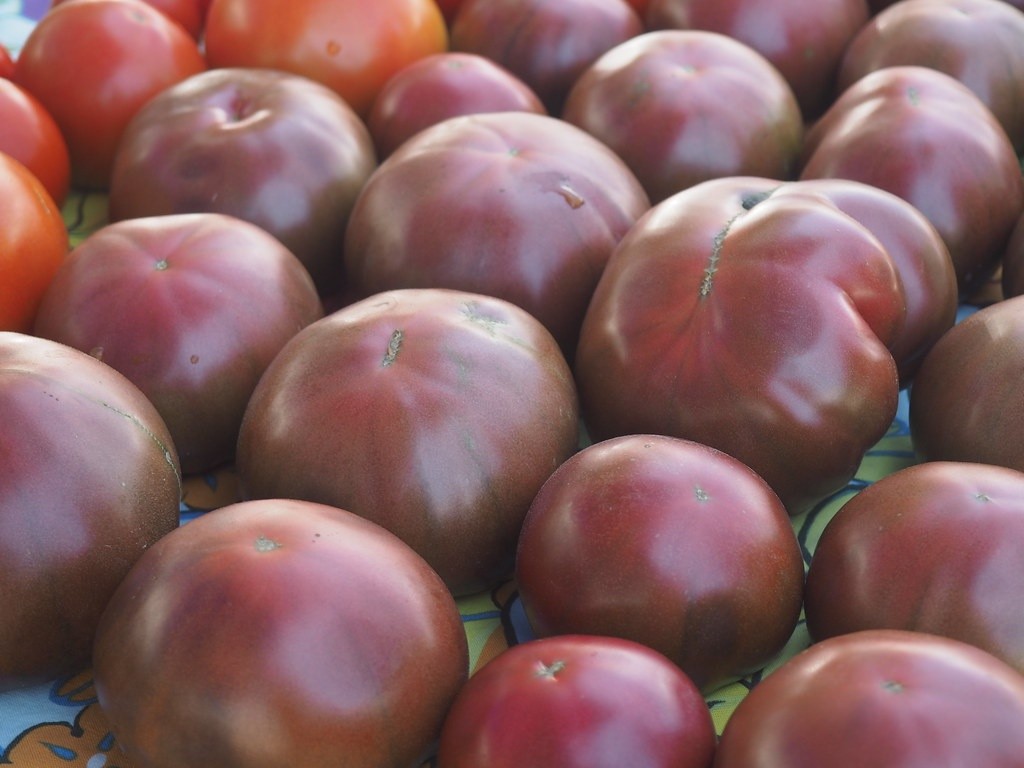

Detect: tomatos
left=0, top=0, right=1024, bottom=768
